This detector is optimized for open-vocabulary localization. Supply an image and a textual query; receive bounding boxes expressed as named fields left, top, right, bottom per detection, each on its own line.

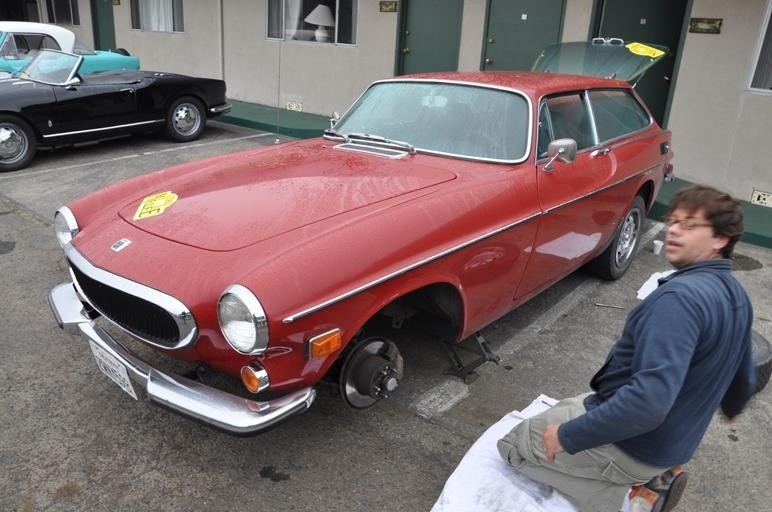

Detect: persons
left=497, top=185, right=757, bottom=512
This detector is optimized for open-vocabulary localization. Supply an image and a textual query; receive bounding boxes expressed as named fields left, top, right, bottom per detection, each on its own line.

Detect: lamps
left=304, top=5, right=335, bottom=42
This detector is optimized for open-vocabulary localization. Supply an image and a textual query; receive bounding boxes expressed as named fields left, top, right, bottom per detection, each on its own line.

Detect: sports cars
left=0, top=47, right=233, bottom=173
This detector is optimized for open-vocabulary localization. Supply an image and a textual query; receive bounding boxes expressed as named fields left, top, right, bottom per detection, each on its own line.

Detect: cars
left=0, top=20, right=140, bottom=82
left=46, top=39, right=674, bottom=440
left=0, top=20, right=76, bottom=56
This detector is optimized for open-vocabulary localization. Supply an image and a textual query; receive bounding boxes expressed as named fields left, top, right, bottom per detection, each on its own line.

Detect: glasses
left=664, top=216, right=714, bottom=231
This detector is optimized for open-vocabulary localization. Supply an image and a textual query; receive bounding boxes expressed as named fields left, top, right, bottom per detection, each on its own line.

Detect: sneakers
left=623, top=466, right=688, bottom=512
